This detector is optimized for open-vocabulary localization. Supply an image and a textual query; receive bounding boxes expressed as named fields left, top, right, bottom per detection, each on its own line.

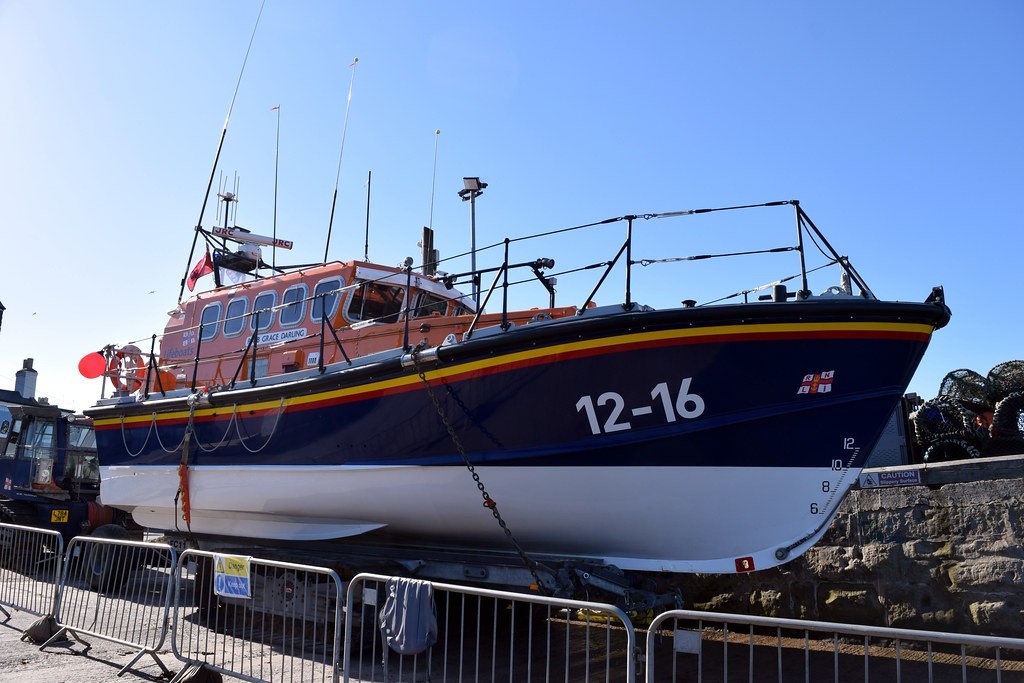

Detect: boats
left=80, top=0, right=958, bottom=574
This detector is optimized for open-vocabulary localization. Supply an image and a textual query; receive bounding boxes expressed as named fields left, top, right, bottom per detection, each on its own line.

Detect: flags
left=185, top=252, right=213, bottom=291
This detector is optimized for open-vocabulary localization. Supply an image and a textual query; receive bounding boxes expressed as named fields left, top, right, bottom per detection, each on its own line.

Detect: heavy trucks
left=0, top=357, right=807, bottom=636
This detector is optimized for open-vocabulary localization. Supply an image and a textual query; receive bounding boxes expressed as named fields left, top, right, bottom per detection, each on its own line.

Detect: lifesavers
left=109, top=347, right=146, bottom=394
